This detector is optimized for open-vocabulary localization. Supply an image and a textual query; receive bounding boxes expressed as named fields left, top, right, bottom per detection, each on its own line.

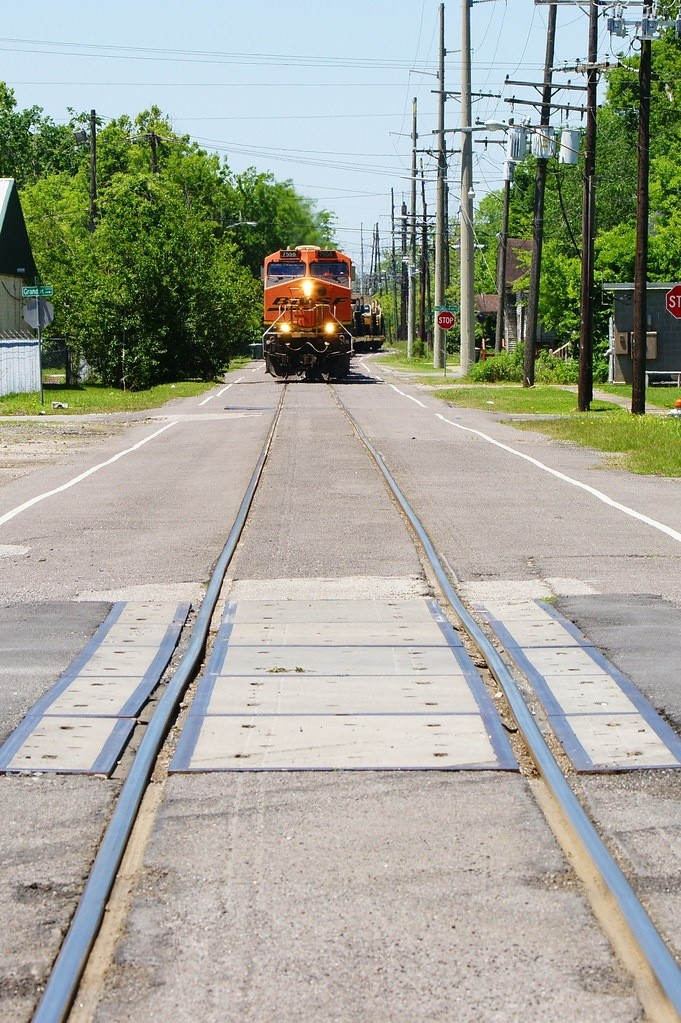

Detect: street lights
left=467, top=186, right=511, bottom=359
left=483, top=116, right=597, bottom=413
left=392, top=214, right=448, bottom=369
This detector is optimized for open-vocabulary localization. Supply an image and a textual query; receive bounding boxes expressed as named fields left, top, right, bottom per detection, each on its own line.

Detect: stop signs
left=665, top=284, right=681, bottom=320
left=437, top=311, right=456, bottom=331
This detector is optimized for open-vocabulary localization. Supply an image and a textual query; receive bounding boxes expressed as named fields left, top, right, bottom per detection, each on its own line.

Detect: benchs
left=645, top=371, right=681, bottom=388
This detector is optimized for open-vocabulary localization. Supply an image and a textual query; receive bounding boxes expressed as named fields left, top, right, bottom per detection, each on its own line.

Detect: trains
left=261, top=245, right=383, bottom=383
